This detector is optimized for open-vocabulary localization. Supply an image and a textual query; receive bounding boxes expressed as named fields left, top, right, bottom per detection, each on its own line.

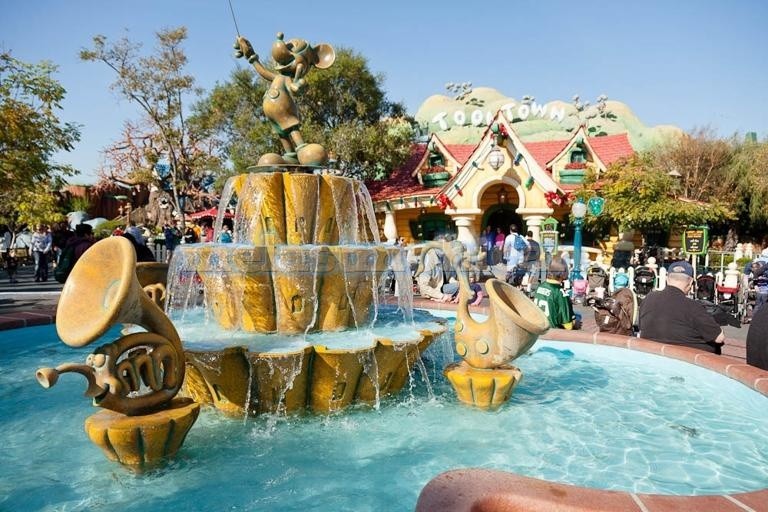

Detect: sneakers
left=9, top=278, right=18, bottom=283
left=34, top=276, right=47, bottom=282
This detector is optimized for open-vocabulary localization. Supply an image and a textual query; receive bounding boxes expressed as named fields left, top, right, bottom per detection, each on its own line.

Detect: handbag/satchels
left=16, top=248, right=27, bottom=258
left=506, top=267, right=523, bottom=285
left=9, top=249, right=17, bottom=258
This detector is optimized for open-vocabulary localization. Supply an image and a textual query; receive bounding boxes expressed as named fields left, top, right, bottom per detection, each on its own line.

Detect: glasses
left=91, top=232, right=94, bottom=235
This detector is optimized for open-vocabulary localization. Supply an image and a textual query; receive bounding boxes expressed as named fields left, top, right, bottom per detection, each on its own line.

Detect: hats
left=668, top=261, right=693, bottom=278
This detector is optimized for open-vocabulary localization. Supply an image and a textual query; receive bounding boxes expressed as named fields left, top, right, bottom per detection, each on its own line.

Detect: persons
left=0, top=217, right=232, bottom=283
left=639, top=262, right=726, bottom=355
left=390, top=236, right=407, bottom=294
left=479, top=223, right=541, bottom=295
left=742, top=262, right=768, bottom=324
left=745, top=297, right=768, bottom=372
left=532, top=257, right=584, bottom=330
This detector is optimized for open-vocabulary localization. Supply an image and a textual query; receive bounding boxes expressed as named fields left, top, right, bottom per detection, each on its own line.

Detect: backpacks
left=54, top=239, right=89, bottom=284
left=513, top=235, right=527, bottom=250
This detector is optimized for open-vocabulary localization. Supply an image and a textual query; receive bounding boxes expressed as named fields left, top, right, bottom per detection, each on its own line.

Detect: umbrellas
left=188, top=207, right=235, bottom=228
